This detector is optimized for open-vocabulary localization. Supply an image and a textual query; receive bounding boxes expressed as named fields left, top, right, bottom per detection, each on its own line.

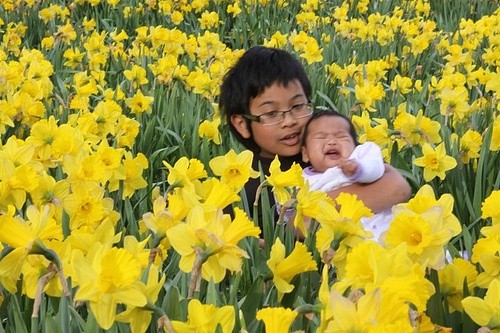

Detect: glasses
left=242, top=103, right=315, bottom=126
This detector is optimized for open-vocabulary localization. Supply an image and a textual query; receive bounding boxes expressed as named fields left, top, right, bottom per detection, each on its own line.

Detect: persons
left=271, top=110, right=405, bottom=246
left=220, top=45, right=412, bottom=250
